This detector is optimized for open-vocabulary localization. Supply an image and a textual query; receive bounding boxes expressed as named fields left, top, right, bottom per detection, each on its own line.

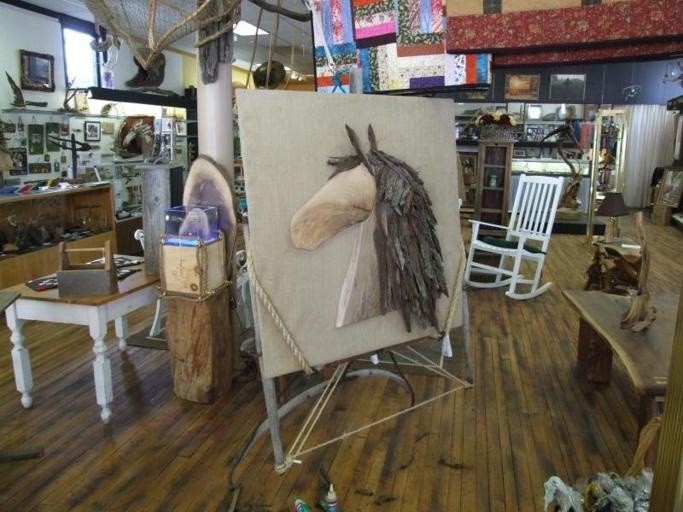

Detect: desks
left=560, top=289, right=680, bottom=467
left=584, top=238, right=643, bottom=294
left=0, top=254, right=165, bottom=423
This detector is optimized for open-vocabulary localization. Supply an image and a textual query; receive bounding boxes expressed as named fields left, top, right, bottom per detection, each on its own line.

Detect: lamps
left=595, top=193, right=628, bottom=242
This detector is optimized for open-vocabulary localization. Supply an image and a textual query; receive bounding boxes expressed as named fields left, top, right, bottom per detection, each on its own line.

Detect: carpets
left=125, top=312, right=170, bottom=351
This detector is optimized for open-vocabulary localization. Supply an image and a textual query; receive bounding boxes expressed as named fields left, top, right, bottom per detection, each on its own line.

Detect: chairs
left=463, top=172, right=568, bottom=300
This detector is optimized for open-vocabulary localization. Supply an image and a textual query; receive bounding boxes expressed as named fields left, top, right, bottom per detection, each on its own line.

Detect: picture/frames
left=654, top=167, right=683, bottom=208
left=85, top=121, right=101, bottom=141
left=456, top=72, right=495, bottom=102
left=548, top=73, right=587, bottom=103
left=20, top=50, right=55, bottom=92
left=503, top=71, right=544, bottom=102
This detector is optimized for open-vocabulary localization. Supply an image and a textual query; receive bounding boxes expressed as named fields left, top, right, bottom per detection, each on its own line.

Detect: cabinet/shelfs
left=0, top=182, right=118, bottom=291
left=455, top=138, right=514, bottom=231
